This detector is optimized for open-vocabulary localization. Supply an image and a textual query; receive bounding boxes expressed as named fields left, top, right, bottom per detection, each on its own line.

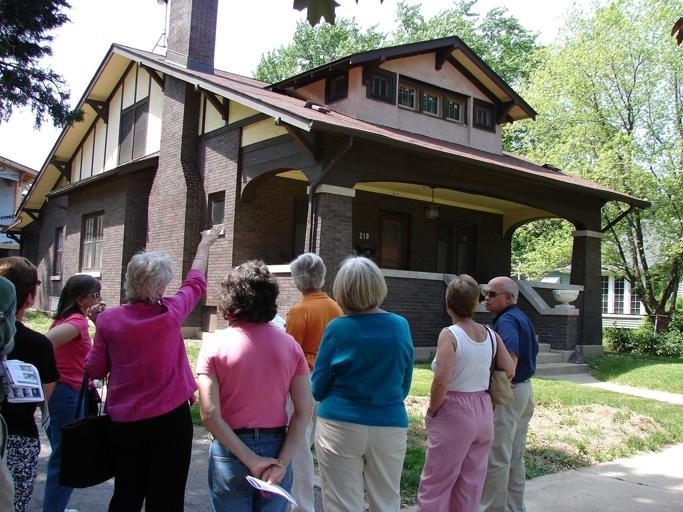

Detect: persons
left=310, top=257, right=415, bottom=512
left=196, top=260, right=315, bottom=512
left=285, top=253, right=344, bottom=512
left=0, top=278, right=17, bottom=512
left=84, top=226, right=222, bottom=512
left=416, top=274, right=515, bottom=512
left=43, top=274, right=103, bottom=512
left=480, top=277, right=539, bottom=512
left=0, top=256, right=61, bottom=512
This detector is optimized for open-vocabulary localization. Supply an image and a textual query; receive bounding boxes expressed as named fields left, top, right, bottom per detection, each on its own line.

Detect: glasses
left=484, top=291, right=506, bottom=298
left=83, top=292, right=101, bottom=298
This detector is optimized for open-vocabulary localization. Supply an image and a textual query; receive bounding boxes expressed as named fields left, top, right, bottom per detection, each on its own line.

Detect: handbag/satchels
left=479, top=324, right=515, bottom=406
left=59, top=361, right=112, bottom=490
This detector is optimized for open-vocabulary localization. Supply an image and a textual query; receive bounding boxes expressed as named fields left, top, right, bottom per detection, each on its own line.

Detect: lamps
left=422, top=188, right=444, bottom=219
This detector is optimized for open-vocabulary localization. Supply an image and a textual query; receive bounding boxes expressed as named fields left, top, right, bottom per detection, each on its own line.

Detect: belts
left=229, top=425, right=283, bottom=437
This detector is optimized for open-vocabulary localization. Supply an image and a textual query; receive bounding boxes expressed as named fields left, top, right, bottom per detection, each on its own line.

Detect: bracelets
left=429, top=408, right=435, bottom=415
